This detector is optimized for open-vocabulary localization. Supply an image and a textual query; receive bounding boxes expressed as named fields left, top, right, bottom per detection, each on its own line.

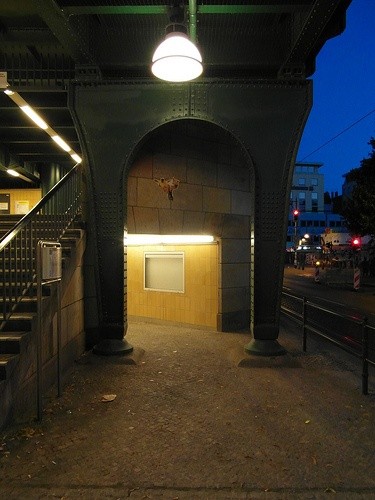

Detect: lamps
left=4, top=87, right=82, bottom=165
left=1, top=166, right=30, bottom=183
left=150, top=33, right=203, bottom=82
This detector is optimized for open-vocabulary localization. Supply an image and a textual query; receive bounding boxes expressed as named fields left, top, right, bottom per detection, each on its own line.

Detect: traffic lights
left=352, top=236, right=361, bottom=254
left=294, top=209, right=298, bottom=221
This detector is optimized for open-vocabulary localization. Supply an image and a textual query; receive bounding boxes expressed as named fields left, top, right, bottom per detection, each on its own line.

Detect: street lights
left=302, top=233, right=326, bottom=270
